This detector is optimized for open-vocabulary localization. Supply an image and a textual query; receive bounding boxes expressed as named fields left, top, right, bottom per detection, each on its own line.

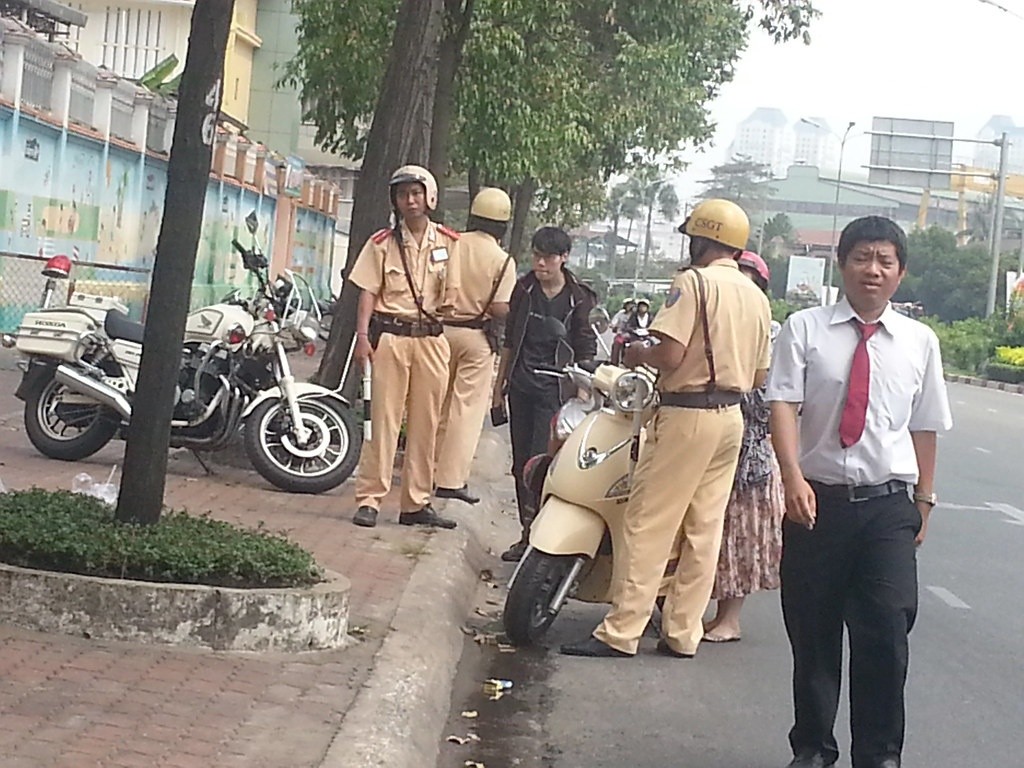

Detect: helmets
left=678, top=199, right=749, bottom=250
left=622, top=297, right=635, bottom=308
left=732, top=250, right=769, bottom=289
left=637, top=298, right=651, bottom=309
left=389, top=165, right=437, bottom=212
left=470, top=187, right=510, bottom=222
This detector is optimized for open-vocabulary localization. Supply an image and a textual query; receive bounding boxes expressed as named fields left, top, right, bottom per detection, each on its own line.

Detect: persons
left=559, top=199, right=772, bottom=659
left=702, top=250, right=788, bottom=643
left=491, top=226, right=597, bottom=563
left=348, top=165, right=460, bottom=529
left=764, top=216, right=954, bottom=768
left=432, top=187, right=518, bottom=504
left=608, top=297, right=655, bottom=367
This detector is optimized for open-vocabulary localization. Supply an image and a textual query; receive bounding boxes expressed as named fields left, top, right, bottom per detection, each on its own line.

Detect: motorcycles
left=0, top=210, right=363, bottom=495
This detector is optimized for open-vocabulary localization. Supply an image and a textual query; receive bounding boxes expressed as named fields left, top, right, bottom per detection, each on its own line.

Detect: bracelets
left=358, top=333, right=368, bottom=336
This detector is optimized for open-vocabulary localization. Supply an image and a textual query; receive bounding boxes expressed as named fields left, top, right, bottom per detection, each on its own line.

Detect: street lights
left=798, top=117, right=858, bottom=306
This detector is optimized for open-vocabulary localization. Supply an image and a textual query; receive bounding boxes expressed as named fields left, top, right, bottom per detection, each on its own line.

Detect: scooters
left=503, top=306, right=684, bottom=647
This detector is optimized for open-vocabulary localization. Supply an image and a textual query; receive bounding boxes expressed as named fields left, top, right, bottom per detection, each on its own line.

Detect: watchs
left=913, top=492, right=937, bottom=506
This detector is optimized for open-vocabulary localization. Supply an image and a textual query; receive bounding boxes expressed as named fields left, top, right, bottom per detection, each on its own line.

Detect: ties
left=839, top=322, right=882, bottom=448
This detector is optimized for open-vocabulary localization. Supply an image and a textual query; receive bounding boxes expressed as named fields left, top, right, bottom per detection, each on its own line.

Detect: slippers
left=702, top=632, right=741, bottom=643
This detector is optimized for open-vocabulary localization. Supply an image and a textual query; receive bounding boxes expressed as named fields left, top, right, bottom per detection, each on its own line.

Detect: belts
left=808, top=479, right=906, bottom=502
left=376, top=317, right=445, bottom=338
left=659, top=391, right=742, bottom=406
left=444, top=319, right=484, bottom=330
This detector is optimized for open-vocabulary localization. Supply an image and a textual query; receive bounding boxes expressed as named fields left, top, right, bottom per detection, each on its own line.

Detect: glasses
left=531, top=252, right=560, bottom=261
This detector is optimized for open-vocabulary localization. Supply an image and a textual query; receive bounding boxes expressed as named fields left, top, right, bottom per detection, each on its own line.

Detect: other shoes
left=399, top=502, right=458, bottom=529
left=560, top=636, right=634, bottom=657
left=783, top=754, right=835, bottom=768
left=434, top=485, right=481, bottom=503
left=353, top=506, right=377, bottom=527
left=658, top=639, right=694, bottom=658
left=500, top=530, right=531, bottom=561
